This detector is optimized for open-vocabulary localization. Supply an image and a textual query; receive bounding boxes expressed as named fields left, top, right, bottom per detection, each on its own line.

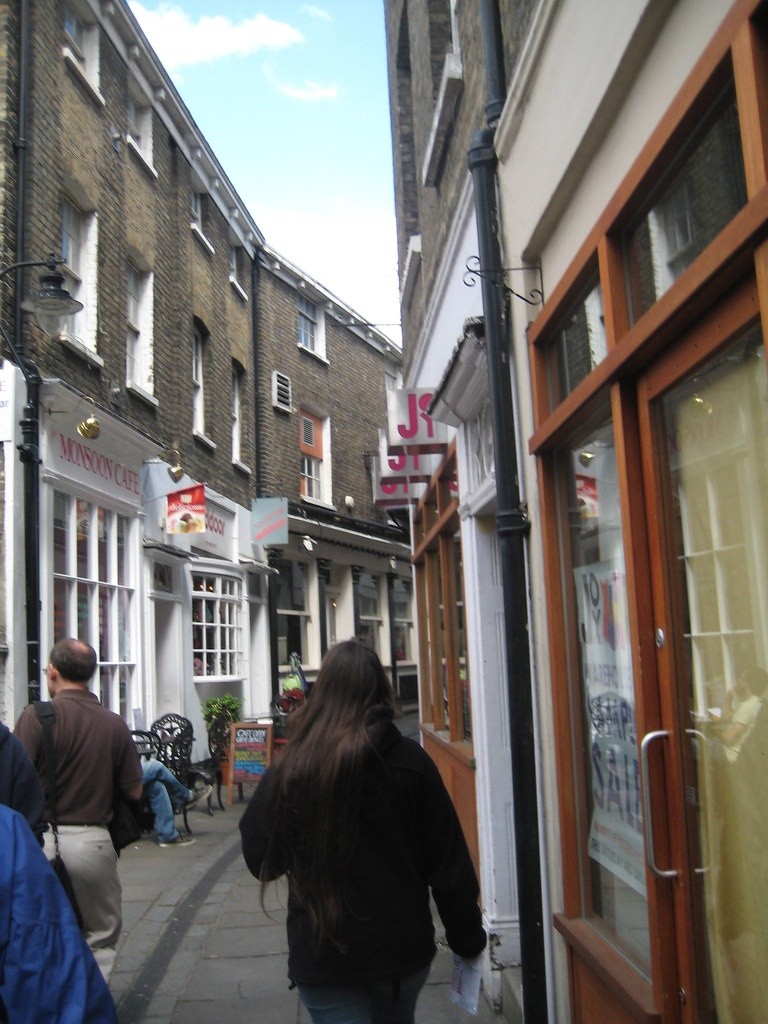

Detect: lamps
left=142, top=450, right=184, bottom=484
left=48, top=395, right=100, bottom=440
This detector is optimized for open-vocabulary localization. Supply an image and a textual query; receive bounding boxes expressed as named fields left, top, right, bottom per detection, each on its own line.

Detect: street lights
left=0, top=253, right=84, bottom=707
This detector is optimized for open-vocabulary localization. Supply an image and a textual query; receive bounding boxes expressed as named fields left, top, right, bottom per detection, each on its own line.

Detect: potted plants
left=201, top=692, right=241, bottom=785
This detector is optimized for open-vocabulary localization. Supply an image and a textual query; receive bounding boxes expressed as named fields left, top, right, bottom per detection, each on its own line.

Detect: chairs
left=132, top=708, right=243, bottom=837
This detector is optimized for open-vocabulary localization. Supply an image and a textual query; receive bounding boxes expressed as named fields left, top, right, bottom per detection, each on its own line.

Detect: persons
left=140, top=760, right=213, bottom=848
left=12, top=637, right=143, bottom=987
left=239, top=641, right=487, bottom=1024
left=0, top=721, right=119, bottom=1024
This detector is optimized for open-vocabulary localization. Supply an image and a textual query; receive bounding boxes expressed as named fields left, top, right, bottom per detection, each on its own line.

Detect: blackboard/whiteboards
left=227, top=723, right=272, bottom=782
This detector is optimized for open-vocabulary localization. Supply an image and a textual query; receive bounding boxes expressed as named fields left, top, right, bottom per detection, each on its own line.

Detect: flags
left=167, top=484, right=206, bottom=534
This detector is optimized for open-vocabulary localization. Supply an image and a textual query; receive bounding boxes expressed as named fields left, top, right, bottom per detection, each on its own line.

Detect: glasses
left=43, top=668, right=49, bottom=675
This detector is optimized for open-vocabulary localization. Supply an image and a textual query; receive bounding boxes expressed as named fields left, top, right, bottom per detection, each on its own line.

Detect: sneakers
left=158, top=833, right=197, bottom=847
left=182, top=784, right=213, bottom=809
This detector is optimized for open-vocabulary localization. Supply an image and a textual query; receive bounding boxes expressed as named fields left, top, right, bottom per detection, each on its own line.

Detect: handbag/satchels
left=50, top=856, right=84, bottom=929
